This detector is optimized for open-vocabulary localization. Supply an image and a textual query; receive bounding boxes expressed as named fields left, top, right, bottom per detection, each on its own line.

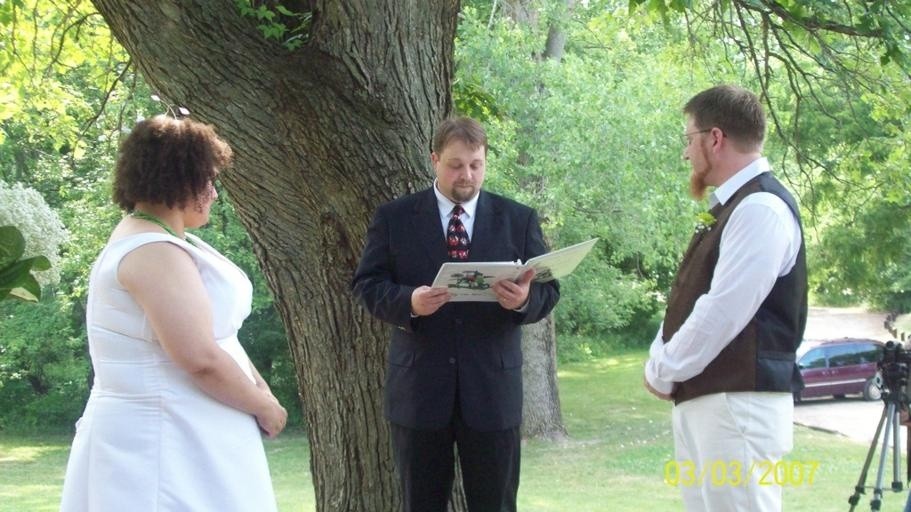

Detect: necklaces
left=132, top=209, right=198, bottom=248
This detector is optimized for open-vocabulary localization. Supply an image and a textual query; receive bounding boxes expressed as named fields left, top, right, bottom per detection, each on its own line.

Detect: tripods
left=849, top=388, right=911, bottom=512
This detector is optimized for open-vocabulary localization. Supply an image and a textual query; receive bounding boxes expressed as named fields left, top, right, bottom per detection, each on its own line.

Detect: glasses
left=679, top=127, right=729, bottom=147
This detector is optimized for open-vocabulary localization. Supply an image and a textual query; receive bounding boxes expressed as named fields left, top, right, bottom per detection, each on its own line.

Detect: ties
left=444, top=203, right=472, bottom=262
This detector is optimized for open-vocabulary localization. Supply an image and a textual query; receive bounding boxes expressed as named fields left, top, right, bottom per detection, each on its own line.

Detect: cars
left=797, top=337, right=889, bottom=401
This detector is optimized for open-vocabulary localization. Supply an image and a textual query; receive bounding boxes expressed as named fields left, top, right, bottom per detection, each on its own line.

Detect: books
left=432, top=236, right=602, bottom=305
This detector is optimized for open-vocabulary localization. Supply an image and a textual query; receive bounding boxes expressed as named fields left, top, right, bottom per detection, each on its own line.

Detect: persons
left=55, top=110, right=292, bottom=512
left=346, top=114, right=564, bottom=512
left=641, top=81, right=813, bottom=510
